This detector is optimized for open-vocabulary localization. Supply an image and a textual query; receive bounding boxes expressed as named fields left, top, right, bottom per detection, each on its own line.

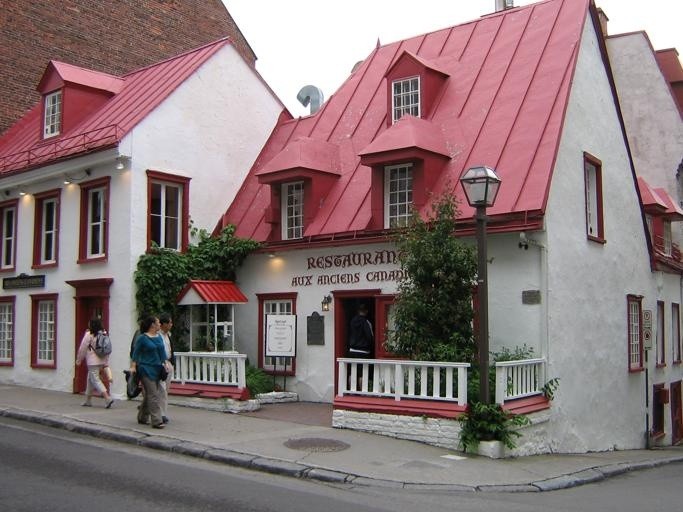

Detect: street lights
left=461, top=166, right=501, bottom=438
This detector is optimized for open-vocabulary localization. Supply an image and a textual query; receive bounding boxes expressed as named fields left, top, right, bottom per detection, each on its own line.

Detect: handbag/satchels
left=127, top=375, right=142, bottom=398
left=160, top=365, right=168, bottom=381
left=102, top=366, right=113, bottom=382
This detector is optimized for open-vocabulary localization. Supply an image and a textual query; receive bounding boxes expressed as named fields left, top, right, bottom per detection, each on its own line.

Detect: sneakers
left=138, top=405, right=169, bottom=428
left=80, top=399, right=114, bottom=409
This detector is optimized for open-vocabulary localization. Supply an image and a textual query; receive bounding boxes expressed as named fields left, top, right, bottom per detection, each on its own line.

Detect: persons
left=348, top=303, right=375, bottom=390
left=129, top=313, right=174, bottom=428
left=76, top=317, right=113, bottom=408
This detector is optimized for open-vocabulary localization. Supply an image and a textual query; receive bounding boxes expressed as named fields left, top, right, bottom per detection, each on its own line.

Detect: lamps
left=115, top=160, right=124, bottom=170
left=17, top=186, right=26, bottom=196
left=63, top=168, right=90, bottom=186
left=320, top=295, right=331, bottom=311
left=265, top=252, right=276, bottom=258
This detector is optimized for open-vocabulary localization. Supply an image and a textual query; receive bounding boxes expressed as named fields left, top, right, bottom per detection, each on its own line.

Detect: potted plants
left=455, top=399, right=532, bottom=460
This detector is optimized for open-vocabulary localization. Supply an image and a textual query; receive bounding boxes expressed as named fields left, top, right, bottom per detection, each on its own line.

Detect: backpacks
left=88, top=330, right=112, bottom=358
